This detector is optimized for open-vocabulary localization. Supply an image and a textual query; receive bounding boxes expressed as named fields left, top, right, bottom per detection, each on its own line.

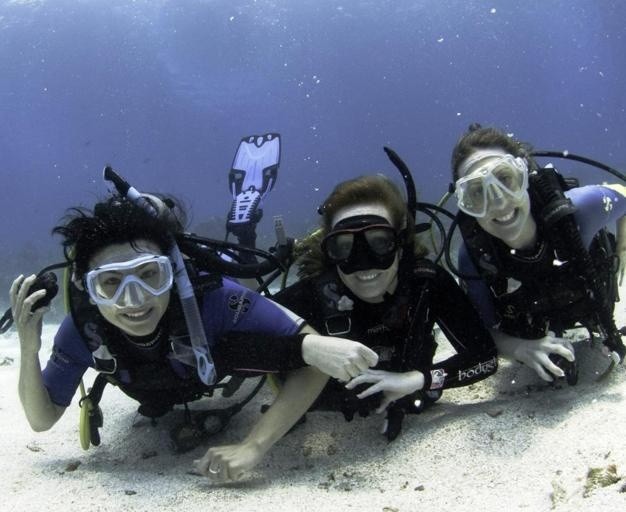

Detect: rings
left=208, top=468, right=221, bottom=474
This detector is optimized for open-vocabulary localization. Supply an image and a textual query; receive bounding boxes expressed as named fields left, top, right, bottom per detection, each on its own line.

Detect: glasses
left=84, top=253, right=175, bottom=306
left=454, top=154, right=528, bottom=218
left=320, top=215, right=397, bottom=275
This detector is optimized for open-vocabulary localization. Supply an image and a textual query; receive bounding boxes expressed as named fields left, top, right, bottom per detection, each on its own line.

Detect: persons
left=447, top=122, right=626, bottom=383
left=7, top=188, right=332, bottom=484
left=211, top=171, right=499, bottom=416
